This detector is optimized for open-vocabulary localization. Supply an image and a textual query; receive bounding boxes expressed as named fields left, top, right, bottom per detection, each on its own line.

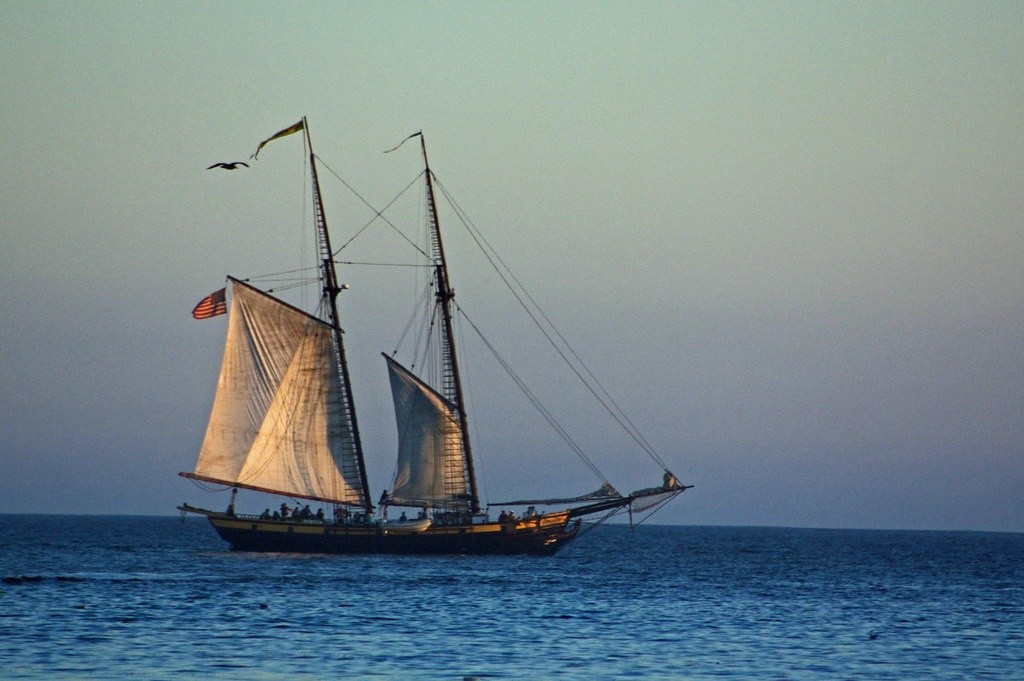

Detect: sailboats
left=176, top=116, right=697, bottom=555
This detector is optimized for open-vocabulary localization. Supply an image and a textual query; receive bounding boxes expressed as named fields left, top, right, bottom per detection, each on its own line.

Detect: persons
left=260, top=502, right=323, bottom=523
left=399, top=512, right=407, bottom=521
left=498, top=510, right=515, bottom=522
